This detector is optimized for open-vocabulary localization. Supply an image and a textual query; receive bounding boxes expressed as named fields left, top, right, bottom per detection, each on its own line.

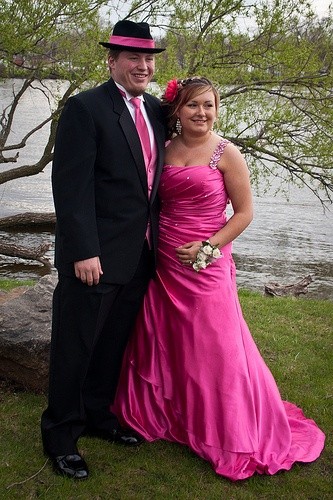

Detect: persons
left=110, top=76, right=325, bottom=480
left=41, top=19, right=231, bottom=480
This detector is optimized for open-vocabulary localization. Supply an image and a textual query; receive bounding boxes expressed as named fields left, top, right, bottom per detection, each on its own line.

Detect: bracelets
left=192, top=238, right=222, bottom=272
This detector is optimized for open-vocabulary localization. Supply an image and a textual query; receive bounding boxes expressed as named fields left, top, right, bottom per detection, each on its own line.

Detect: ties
left=116, top=87, right=151, bottom=162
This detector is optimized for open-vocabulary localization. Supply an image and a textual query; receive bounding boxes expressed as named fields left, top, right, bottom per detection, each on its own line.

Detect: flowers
left=165, top=78, right=179, bottom=102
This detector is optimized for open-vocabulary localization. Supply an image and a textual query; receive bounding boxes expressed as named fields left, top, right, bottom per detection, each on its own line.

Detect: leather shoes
left=87, top=425, right=143, bottom=447
left=53, top=455, right=88, bottom=480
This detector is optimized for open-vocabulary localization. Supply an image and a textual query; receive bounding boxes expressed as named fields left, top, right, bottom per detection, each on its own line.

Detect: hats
left=98, top=20, right=166, bottom=54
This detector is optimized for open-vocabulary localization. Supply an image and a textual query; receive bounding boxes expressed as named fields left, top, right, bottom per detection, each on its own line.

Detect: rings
left=189, top=260, right=192, bottom=263
